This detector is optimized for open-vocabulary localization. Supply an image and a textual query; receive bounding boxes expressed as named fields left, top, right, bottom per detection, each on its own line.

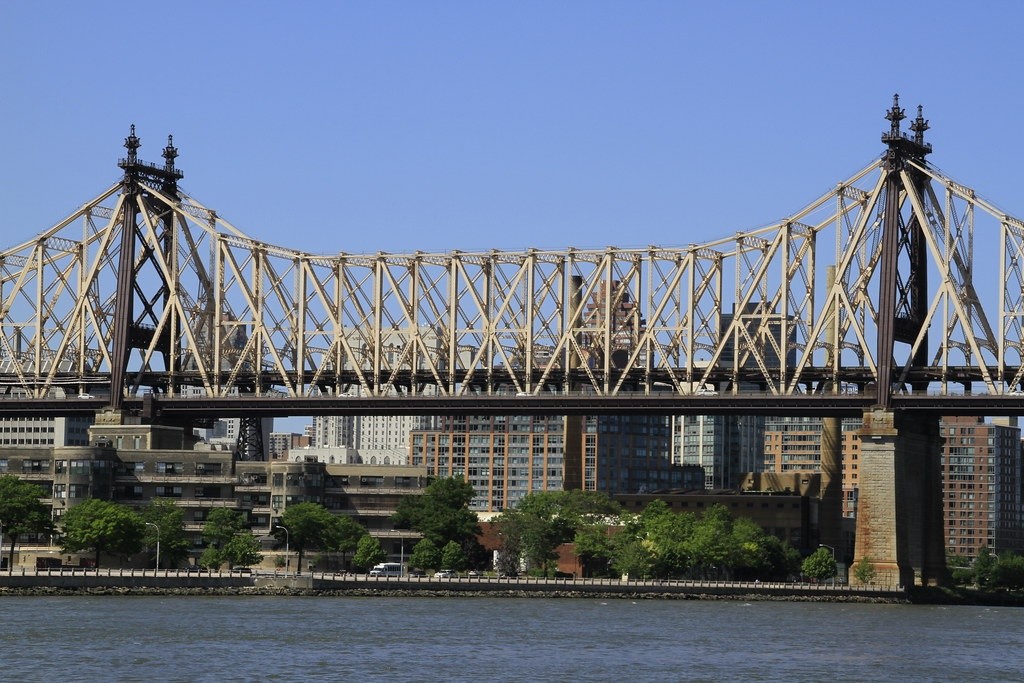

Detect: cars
left=228, top=567, right=253, bottom=574
left=469, top=568, right=483, bottom=576
left=182, top=564, right=208, bottom=573
left=434, top=570, right=457, bottom=578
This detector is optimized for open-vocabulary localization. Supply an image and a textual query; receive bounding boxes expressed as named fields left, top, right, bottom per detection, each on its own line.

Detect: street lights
left=276, top=524, right=288, bottom=578
left=989, top=553, right=1000, bottom=565
left=390, top=529, right=404, bottom=569
left=145, top=522, right=160, bottom=573
left=819, top=543, right=835, bottom=588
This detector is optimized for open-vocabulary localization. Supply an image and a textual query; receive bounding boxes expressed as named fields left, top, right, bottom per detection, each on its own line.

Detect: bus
left=369, top=563, right=405, bottom=576
